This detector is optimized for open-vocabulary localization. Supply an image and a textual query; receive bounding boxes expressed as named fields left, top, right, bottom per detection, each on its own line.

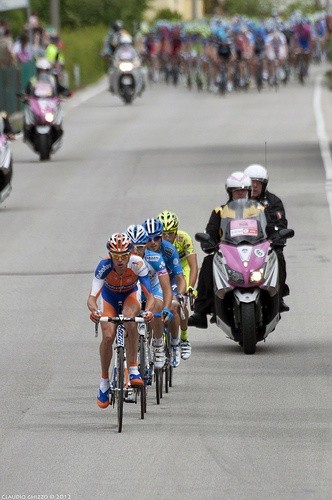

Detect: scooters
left=194, top=199, right=295, bottom=356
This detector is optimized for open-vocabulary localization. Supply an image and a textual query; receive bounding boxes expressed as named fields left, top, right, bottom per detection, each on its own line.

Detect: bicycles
left=95, top=288, right=195, bottom=434
left=140, top=35, right=320, bottom=95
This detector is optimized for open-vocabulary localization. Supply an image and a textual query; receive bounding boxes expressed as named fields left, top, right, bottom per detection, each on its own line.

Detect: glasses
left=110, top=252, right=129, bottom=260
left=148, top=236, right=160, bottom=242
left=163, top=230, right=176, bottom=237
left=132, top=245, right=146, bottom=250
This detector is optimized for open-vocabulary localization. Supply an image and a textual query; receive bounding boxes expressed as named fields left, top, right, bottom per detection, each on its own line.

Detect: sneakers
left=129, top=364, right=144, bottom=387
left=167, top=340, right=181, bottom=367
left=152, top=340, right=166, bottom=369
left=180, top=339, right=192, bottom=361
left=96, top=379, right=110, bottom=409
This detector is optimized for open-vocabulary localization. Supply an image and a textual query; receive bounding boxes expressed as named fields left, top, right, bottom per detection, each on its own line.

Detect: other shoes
left=187, top=313, right=208, bottom=329
left=280, top=300, right=290, bottom=312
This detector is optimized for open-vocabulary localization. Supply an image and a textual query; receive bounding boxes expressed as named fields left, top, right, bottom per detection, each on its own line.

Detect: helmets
left=34, top=57, right=51, bottom=69
left=224, top=171, right=252, bottom=192
left=143, top=219, right=164, bottom=237
left=156, top=211, right=180, bottom=232
left=126, top=224, right=149, bottom=243
left=106, top=232, right=134, bottom=253
left=243, top=163, right=270, bottom=183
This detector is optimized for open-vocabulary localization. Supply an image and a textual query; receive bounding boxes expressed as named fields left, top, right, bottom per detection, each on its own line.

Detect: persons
left=135, top=10, right=332, bottom=91
left=104, top=21, right=133, bottom=59
left=187, top=164, right=291, bottom=329
left=87, top=211, right=198, bottom=409
left=0, top=12, right=73, bottom=140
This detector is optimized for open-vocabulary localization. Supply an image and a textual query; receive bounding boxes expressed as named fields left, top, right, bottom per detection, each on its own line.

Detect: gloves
left=270, top=234, right=280, bottom=245
left=204, top=242, right=215, bottom=252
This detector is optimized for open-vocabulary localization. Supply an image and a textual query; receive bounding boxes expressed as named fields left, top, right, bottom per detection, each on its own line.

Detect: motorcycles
left=15, top=73, right=71, bottom=162
left=0, top=116, right=20, bottom=203
left=104, top=34, right=147, bottom=105
